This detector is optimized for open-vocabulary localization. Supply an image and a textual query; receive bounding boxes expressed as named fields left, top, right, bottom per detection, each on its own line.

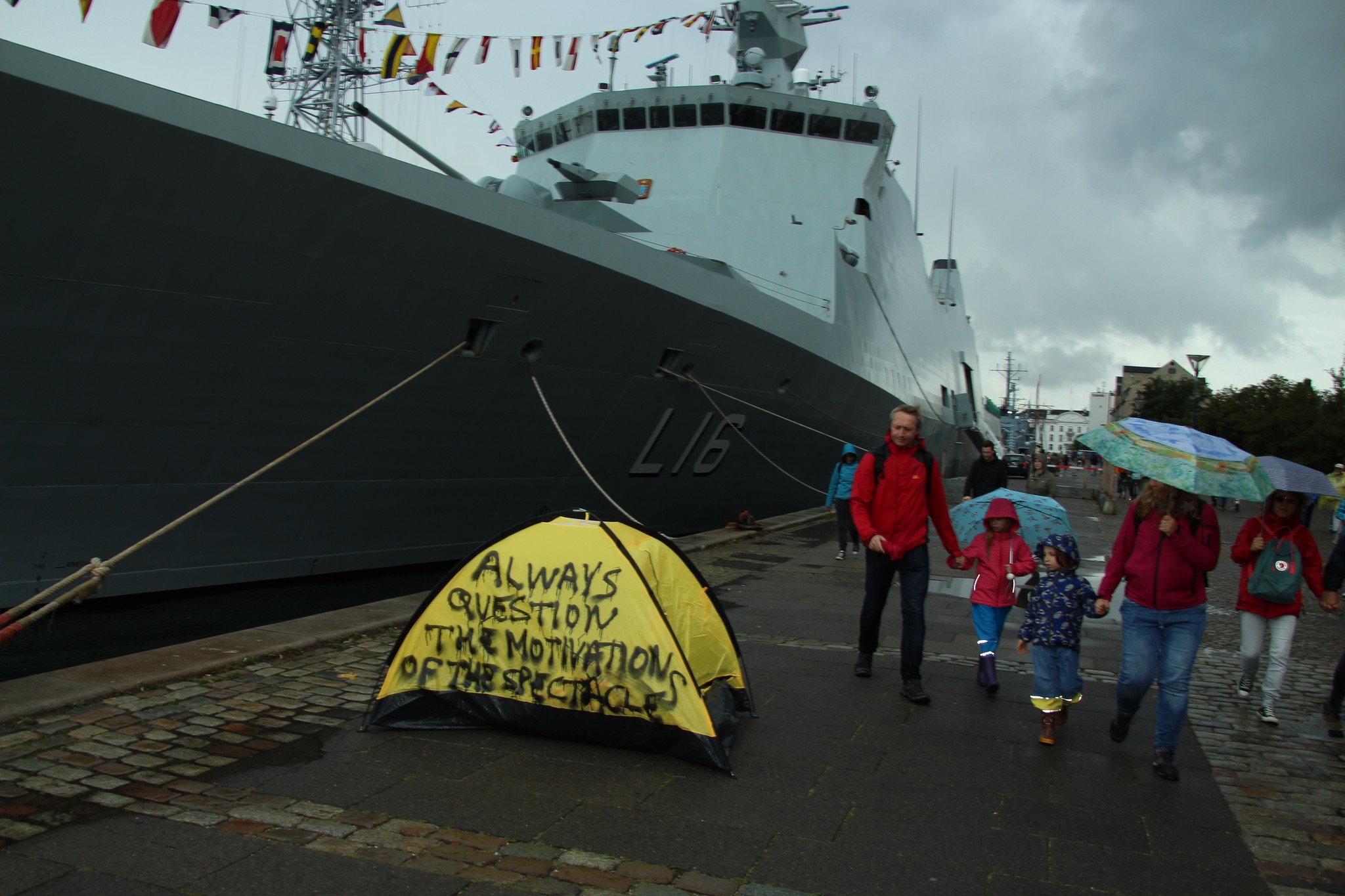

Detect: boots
left=978, top=654, right=1000, bottom=692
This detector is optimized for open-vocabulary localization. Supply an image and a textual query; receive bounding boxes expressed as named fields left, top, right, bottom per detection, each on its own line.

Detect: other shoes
left=1040, top=712, right=1057, bottom=745
left=1153, top=750, right=1180, bottom=779
left=1110, top=709, right=1131, bottom=742
left=1058, top=704, right=1069, bottom=726
left=1322, top=701, right=1343, bottom=729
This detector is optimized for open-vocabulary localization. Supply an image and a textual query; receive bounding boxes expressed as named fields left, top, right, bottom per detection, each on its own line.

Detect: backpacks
left=1246, top=516, right=1303, bottom=604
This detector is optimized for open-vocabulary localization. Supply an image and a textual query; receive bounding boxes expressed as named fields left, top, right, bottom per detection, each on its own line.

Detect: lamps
left=521, top=106, right=533, bottom=120
left=598, top=82, right=610, bottom=92
left=709, top=75, right=720, bottom=85
left=865, top=85, right=878, bottom=102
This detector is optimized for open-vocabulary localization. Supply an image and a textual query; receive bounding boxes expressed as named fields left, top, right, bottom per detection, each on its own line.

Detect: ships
left=0, top=2, right=1007, bottom=622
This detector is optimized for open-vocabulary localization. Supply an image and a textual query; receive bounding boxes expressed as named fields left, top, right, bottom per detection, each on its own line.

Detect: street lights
left=1183, top=354, right=1210, bottom=430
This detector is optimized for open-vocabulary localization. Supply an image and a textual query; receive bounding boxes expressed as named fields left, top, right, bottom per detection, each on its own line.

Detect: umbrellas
left=933, top=487, right=1079, bottom=554
left=1075, top=417, right=1276, bottom=518
left=1251, top=456, right=1340, bottom=539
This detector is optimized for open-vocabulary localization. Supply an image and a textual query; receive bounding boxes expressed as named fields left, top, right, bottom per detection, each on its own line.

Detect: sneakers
left=852, top=542, right=859, bottom=555
left=852, top=651, right=875, bottom=676
left=1237, top=674, right=1253, bottom=698
left=1256, top=704, right=1280, bottom=724
left=900, top=679, right=930, bottom=703
left=835, top=550, right=846, bottom=560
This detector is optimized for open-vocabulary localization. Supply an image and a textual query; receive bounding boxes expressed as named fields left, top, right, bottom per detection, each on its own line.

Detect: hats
left=1335, top=464, right=1345, bottom=469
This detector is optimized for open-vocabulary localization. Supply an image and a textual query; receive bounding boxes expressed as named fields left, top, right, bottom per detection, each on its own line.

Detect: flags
left=265, top=19, right=295, bottom=77
left=401, top=8, right=716, bottom=147
left=207, top=5, right=242, bottom=29
left=357, top=2, right=406, bottom=65
left=381, top=33, right=411, bottom=79
left=142, top=0, right=184, bottom=49
left=302, top=22, right=328, bottom=63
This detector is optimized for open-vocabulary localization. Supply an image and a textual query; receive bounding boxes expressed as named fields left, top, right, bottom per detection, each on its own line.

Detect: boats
left=987, top=351, right=1037, bottom=459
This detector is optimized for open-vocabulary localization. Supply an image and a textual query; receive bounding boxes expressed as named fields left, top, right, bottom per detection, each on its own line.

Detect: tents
left=357, top=513, right=759, bottom=780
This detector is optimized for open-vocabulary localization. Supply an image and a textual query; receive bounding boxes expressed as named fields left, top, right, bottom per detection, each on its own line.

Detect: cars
left=1001, top=453, right=1030, bottom=480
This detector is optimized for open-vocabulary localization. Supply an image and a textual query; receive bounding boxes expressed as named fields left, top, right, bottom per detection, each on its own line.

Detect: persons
left=1079, top=452, right=1099, bottom=476
left=1114, top=465, right=1150, bottom=502
left=1095, top=478, right=1222, bottom=782
left=1316, top=463, right=1345, bottom=533
left=1015, top=533, right=1109, bottom=744
left=1046, top=452, right=1071, bottom=477
left=1318, top=497, right=1345, bottom=732
left=1231, top=489, right=1343, bottom=723
left=947, top=498, right=1038, bottom=696
left=1025, top=453, right=1056, bottom=499
left=825, top=443, right=859, bottom=561
left=961, top=439, right=1008, bottom=502
left=1210, top=496, right=1242, bottom=513
left=849, top=405, right=964, bottom=705
